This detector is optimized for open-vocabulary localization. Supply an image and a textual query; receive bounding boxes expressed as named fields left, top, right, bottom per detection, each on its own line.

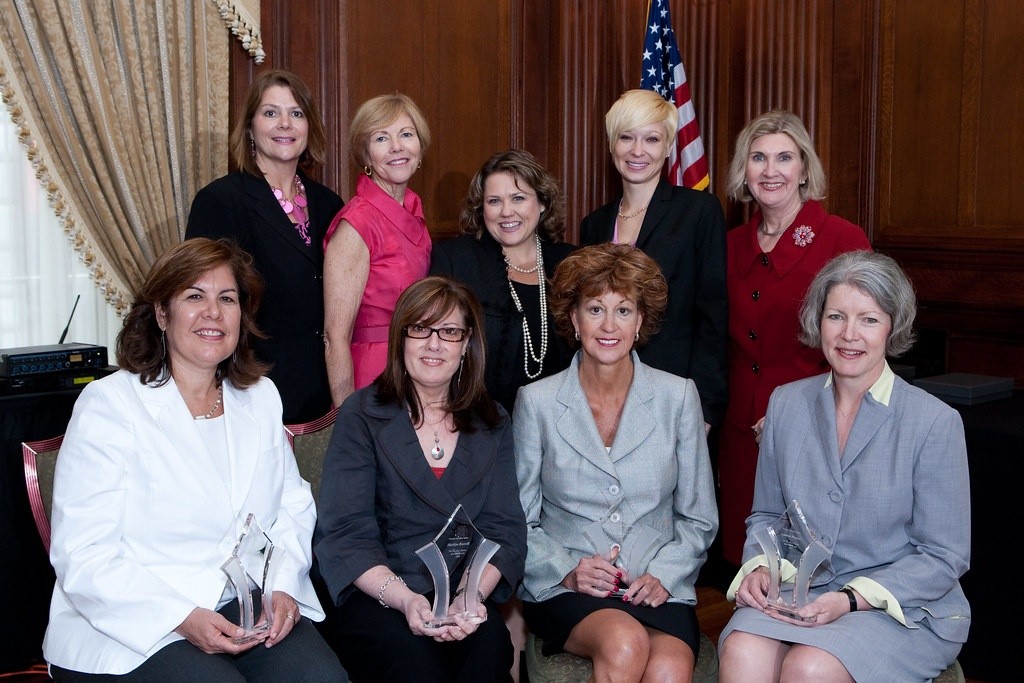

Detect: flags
left=640, top=0, right=710, bottom=191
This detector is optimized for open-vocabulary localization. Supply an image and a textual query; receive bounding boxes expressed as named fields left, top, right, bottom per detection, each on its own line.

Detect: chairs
left=19, top=406, right=966, bottom=683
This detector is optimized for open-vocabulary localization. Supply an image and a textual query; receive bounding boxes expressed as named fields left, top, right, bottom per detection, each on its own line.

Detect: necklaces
left=761, top=221, right=786, bottom=236
left=618, top=197, right=648, bottom=219
left=194, top=385, right=222, bottom=420
left=504, top=234, right=547, bottom=379
left=272, top=174, right=311, bottom=245
left=431, top=431, right=445, bottom=460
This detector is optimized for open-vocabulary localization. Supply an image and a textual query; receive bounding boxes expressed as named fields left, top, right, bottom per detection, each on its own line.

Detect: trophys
left=219, top=513, right=275, bottom=643
left=752, top=499, right=833, bottom=623
left=415, top=504, right=501, bottom=628
left=582, top=494, right=665, bottom=597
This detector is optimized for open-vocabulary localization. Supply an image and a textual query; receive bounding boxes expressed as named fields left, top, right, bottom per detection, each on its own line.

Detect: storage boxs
left=912, top=372, right=1015, bottom=405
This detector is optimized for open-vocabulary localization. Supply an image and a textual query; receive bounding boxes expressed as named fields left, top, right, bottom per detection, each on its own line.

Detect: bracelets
left=453, top=588, right=484, bottom=603
left=378, top=576, right=407, bottom=608
left=841, top=589, right=857, bottom=612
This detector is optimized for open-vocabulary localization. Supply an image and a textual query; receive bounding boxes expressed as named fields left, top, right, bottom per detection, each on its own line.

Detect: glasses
left=402, top=323, right=469, bottom=342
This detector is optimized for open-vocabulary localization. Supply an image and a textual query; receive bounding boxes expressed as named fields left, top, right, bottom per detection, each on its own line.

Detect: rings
left=288, top=616, right=295, bottom=624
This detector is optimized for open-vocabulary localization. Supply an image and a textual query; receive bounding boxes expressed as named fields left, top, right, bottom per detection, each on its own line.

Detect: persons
left=580, top=89, right=728, bottom=423
left=428, top=149, right=580, bottom=414
left=322, top=94, right=431, bottom=408
left=720, top=109, right=874, bottom=565
left=314, top=276, right=527, bottom=683
left=184, top=69, right=345, bottom=426
left=43, top=238, right=349, bottom=683
left=717, top=250, right=972, bottom=683
left=509, top=244, right=719, bottom=683
